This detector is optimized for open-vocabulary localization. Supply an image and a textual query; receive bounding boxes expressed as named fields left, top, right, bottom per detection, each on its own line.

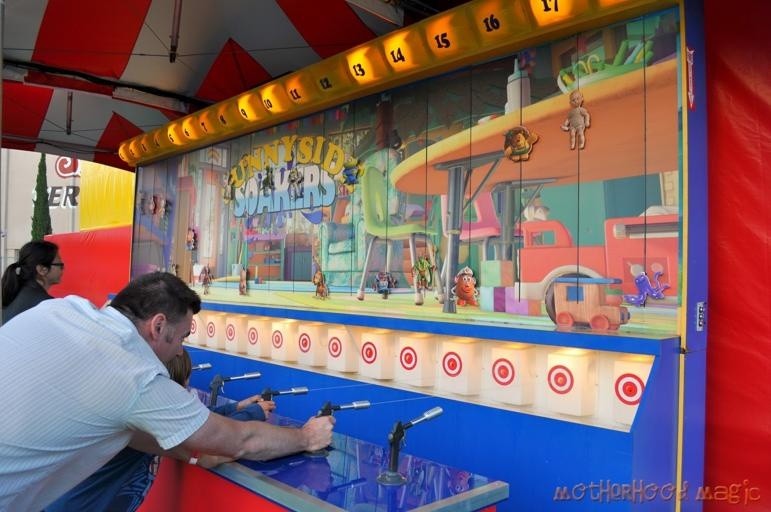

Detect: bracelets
left=187, top=450, right=199, bottom=467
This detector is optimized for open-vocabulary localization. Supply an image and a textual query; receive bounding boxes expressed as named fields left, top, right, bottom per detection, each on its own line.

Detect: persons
left=41, top=346, right=278, bottom=512
left=2, top=240, right=65, bottom=325
left=0, top=270, right=340, bottom=512
